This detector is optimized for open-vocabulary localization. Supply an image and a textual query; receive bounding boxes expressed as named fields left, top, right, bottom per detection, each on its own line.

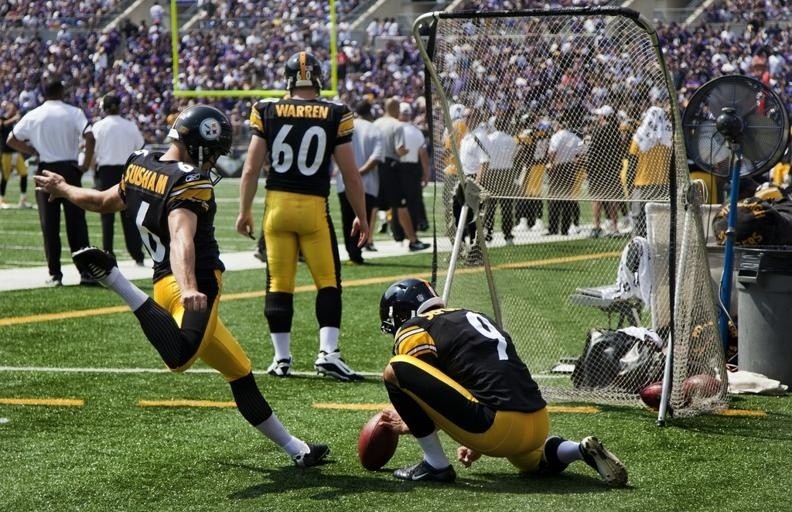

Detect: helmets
left=379, top=278, right=445, bottom=333
left=167, top=105, right=232, bottom=155
left=285, top=52, right=322, bottom=88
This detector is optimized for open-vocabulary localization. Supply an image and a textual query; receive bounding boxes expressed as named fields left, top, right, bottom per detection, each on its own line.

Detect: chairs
left=569, top=236, right=650, bottom=329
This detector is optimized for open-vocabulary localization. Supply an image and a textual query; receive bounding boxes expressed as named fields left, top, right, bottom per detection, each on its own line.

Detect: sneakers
left=266, top=357, right=291, bottom=376
left=44, top=275, right=61, bottom=286
left=71, top=247, right=117, bottom=281
left=314, top=352, right=365, bottom=381
left=363, top=243, right=376, bottom=251
left=394, top=460, right=455, bottom=482
left=293, top=442, right=329, bottom=467
left=586, top=228, right=602, bottom=237
left=602, top=231, right=622, bottom=237
left=579, top=436, right=628, bottom=487
left=409, top=241, right=432, bottom=251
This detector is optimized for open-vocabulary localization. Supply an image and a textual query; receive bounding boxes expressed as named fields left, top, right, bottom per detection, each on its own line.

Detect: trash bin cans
left=734, top=244, right=792, bottom=393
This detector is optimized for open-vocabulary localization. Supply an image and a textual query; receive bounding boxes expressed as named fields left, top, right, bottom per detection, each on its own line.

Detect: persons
left=376, top=276, right=629, bottom=487
left=1, top=1, right=792, bottom=268
left=32, top=105, right=331, bottom=469
left=235, top=50, right=370, bottom=383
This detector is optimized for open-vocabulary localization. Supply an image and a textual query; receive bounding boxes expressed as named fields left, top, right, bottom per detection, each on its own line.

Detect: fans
left=681, top=74, right=792, bottom=358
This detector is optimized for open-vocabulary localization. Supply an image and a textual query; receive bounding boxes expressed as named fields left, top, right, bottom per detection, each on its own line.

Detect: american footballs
left=683, top=375, right=722, bottom=399
left=359, top=412, right=398, bottom=470
left=640, top=382, right=692, bottom=408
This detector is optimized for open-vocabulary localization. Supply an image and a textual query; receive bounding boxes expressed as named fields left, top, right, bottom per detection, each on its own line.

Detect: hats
left=399, top=102, right=411, bottom=114
left=591, top=105, right=615, bottom=116
left=449, top=103, right=471, bottom=119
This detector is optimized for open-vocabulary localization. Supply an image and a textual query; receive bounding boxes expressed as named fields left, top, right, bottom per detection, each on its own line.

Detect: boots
left=530, top=217, right=542, bottom=229
left=512, top=216, right=528, bottom=230
